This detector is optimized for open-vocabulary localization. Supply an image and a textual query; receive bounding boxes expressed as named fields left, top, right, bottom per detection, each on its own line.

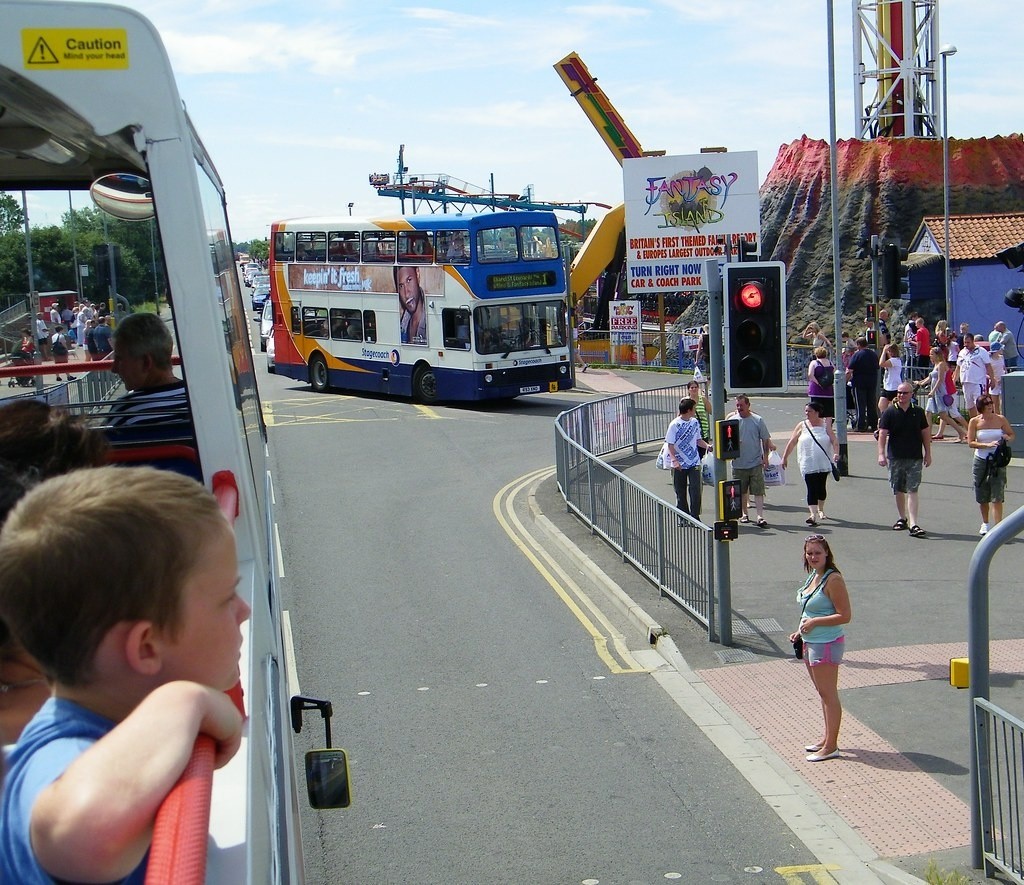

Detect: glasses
left=898, top=391, right=912, bottom=395
left=984, top=401, right=993, bottom=405
left=804, top=534, right=825, bottom=542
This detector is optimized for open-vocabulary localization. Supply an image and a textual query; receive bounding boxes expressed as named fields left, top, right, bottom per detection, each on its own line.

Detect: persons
left=665, top=325, right=776, bottom=527
left=0, top=467, right=252, bottom=885
left=803, top=309, right=1018, bottom=444
left=458, top=315, right=490, bottom=340
left=878, top=382, right=931, bottom=536
left=781, top=402, right=839, bottom=524
left=576, top=344, right=587, bottom=372
left=790, top=534, right=852, bottom=761
left=100, top=313, right=188, bottom=426
left=323, top=309, right=361, bottom=340
left=393, top=265, right=427, bottom=346
left=0, top=299, right=125, bottom=791
left=968, top=395, right=1016, bottom=535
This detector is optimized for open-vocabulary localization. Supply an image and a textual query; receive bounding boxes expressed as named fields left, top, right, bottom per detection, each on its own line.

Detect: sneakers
left=979, top=522, right=989, bottom=535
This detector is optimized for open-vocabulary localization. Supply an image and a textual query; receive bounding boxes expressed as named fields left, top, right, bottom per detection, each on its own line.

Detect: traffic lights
left=721, top=479, right=743, bottom=519
left=722, top=260, right=787, bottom=394
left=900, top=247, right=909, bottom=294
left=867, top=304, right=876, bottom=321
left=718, top=419, right=741, bottom=461
left=867, top=331, right=877, bottom=348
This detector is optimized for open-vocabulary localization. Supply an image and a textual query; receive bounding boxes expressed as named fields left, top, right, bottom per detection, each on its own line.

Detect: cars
left=234, top=248, right=276, bottom=375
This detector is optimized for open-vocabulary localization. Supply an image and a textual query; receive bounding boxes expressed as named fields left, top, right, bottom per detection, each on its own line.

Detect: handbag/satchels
left=793, top=638, right=804, bottom=659
left=943, top=394, right=954, bottom=407
left=831, top=463, right=840, bottom=481
left=693, top=365, right=703, bottom=383
left=656, top=438, right=715, bottom=488
left=762, top=449, right=786, bottom=486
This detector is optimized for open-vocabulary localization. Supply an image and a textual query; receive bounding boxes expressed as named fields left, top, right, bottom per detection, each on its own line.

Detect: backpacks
left=51, top=335, right=68, bottom=359
left=985, top=437, right=1012, bottom=468
left=814, top=360, right=833, bottom=388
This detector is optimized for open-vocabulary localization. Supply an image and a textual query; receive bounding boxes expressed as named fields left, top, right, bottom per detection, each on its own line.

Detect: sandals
left=892, top=518, right=908, bottom=530
left=909, top=525, right=925, bottom=535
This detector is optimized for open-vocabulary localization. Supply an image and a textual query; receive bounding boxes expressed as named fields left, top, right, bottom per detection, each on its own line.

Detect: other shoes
left=853, top=419, right=970, bottom=443
left=67, top=376, right=76, bottom=381
left=805, top=745, right=822, bottom=751
left=582, top=363, right=587, bottom=372
left=677, top=499, right=826, bottom=532
left=806, top=748, right=839, bottom=761
left=57, top=377, right=62, bottom=381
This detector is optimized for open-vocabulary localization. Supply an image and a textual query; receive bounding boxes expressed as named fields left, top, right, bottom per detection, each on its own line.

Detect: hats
left=989, top=342, right=1005, bottom=353
left=52, top=303, right=58, bottom=308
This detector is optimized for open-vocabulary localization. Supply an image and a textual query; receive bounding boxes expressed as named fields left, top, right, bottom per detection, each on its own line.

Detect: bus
left=270, top=209, right=573, bottom=404
left=2, top=0, right=349, bottom=883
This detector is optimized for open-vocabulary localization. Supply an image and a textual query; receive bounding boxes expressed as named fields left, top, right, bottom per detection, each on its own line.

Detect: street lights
left=938, top=45, right=958, bottom=337
